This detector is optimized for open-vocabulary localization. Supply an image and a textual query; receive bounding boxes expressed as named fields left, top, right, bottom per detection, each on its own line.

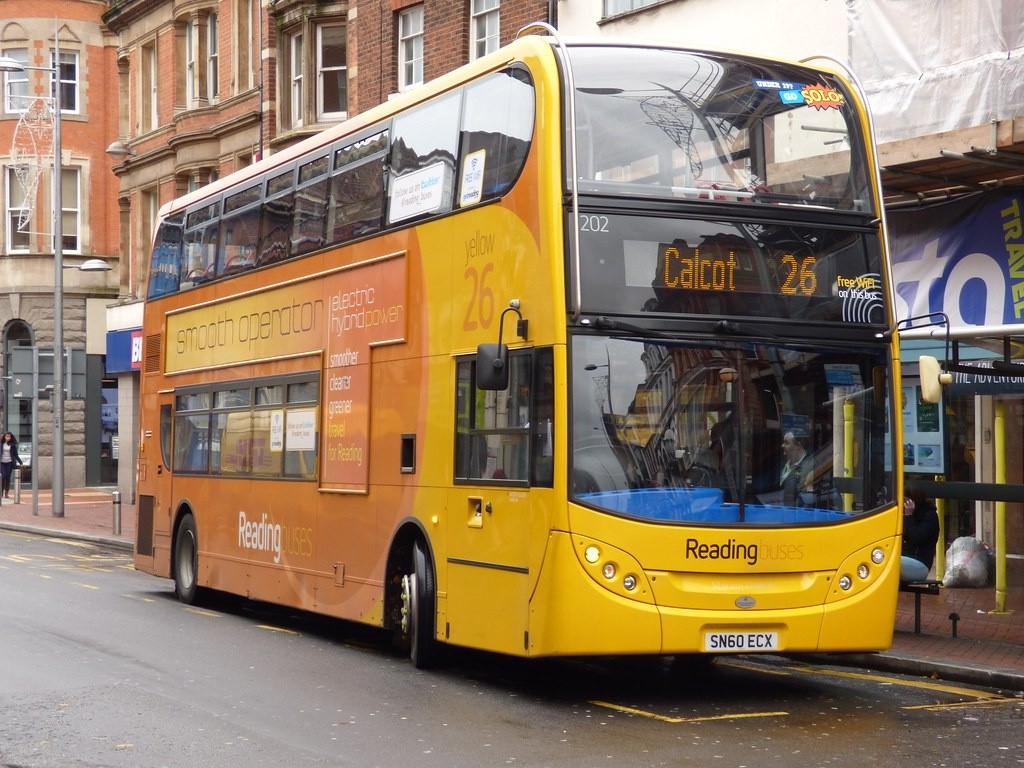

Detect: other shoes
left=5, top=495, right=9, bottom=498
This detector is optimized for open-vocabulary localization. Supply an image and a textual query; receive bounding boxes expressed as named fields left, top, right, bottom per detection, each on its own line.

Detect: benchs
left=900, top=579, right=943, bottom=634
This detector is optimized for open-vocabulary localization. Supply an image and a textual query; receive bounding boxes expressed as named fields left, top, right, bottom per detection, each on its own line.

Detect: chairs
left=185, top=267, right=209, bottom=285
left=224, top=255, right=254, bottom=275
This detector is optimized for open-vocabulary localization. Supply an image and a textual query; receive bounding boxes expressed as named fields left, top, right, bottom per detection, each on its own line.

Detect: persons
left=532, top=397, right=666, bottom=489
left=0, top=432, right=23, bottom=498
left=780, top=430, right=842, bottom=511
left=899, top=474, right=940, bottom=580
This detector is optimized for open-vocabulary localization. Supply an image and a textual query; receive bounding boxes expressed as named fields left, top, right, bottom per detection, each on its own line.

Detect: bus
left=128, top=23, right=954, bottom=659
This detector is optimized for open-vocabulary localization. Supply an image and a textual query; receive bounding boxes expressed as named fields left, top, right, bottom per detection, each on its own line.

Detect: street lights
left=0, top=57, right=111, bottom=516
left=718, top=366, right=746, bottom=521
left=582, top=345, right=615, bottom=427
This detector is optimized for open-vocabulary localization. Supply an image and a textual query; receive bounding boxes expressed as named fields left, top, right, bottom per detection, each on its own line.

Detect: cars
left=16, top=441, right=32, bottom=479
